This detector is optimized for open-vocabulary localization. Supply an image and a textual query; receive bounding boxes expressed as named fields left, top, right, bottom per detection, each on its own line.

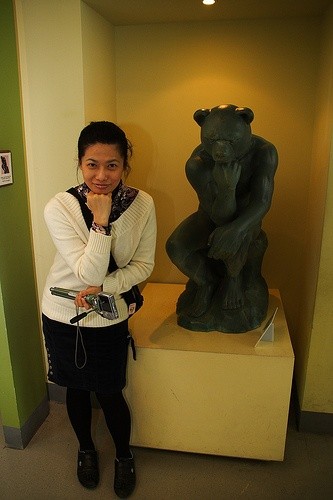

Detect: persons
left=39, top=120, right=158, bottom=500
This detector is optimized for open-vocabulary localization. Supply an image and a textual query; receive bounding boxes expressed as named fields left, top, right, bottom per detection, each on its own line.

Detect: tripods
left=50, top=286, right=97, bottom=324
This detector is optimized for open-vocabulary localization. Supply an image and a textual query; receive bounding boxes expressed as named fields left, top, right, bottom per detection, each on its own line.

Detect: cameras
left=97, top=292, right=118, bottom=320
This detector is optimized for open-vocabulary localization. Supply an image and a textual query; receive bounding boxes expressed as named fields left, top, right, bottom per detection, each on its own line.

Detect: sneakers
left=77, top=440, right=100, bottom=489
left=113, top=450, right=136, bottom=498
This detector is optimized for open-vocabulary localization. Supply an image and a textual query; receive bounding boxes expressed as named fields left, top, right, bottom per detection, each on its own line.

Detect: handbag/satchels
left=66, top=186, right=144, bottom=316
